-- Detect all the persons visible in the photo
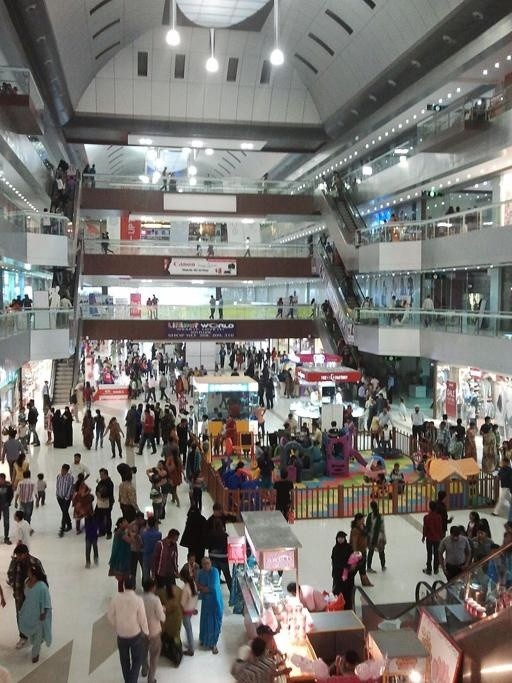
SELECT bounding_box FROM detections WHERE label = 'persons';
[244,236,252,256]
[207,236,215,257]
[309,298,350,362]
[7,294,33,326]
[361,297,407,325]
[160,166,170,192]
[195,233,203,255]
[307,158,486,297]
[147,294,159,320]
[209,295,223,320]
[1,340,511,682]
[260,171,269,194]
[60,291,73,324]
[421,294,434,327]
[2,77,114,287]
[275,295,294,318]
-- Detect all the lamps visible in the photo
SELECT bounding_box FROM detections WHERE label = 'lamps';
[203,26,222,74]
[268,0,286,67]
[162,0,184,48]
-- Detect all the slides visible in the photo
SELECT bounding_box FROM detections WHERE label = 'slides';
[351,449,367,468]
